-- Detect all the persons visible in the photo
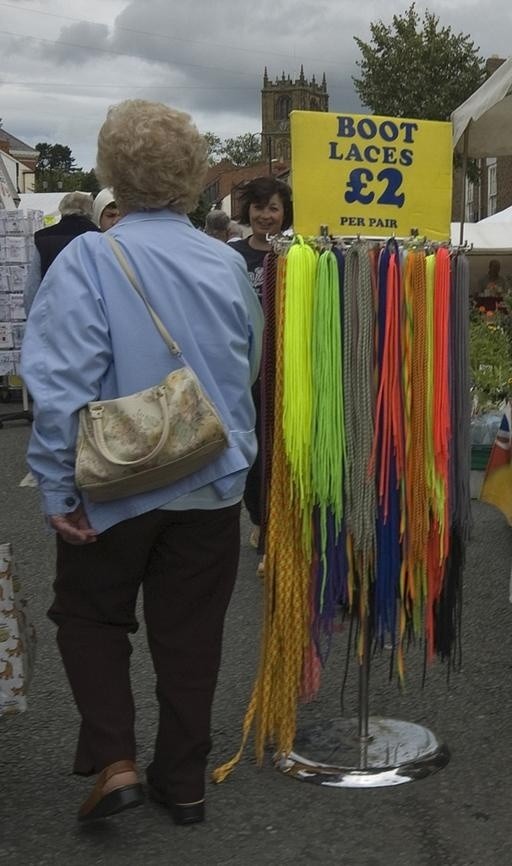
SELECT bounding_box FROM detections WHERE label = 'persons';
[187,195,243,246]
[223,174,297,581]
[473,258,505,305]
[89,186,120,232]
[16,96,260,833]
[22,192,100,320]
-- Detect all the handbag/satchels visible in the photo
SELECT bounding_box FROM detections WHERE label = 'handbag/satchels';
[75,368,229,506]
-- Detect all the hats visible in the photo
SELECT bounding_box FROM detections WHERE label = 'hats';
[92,187,115,229]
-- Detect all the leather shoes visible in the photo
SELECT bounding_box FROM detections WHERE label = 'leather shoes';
[137,770,207,826]
[78,760,144,823]
